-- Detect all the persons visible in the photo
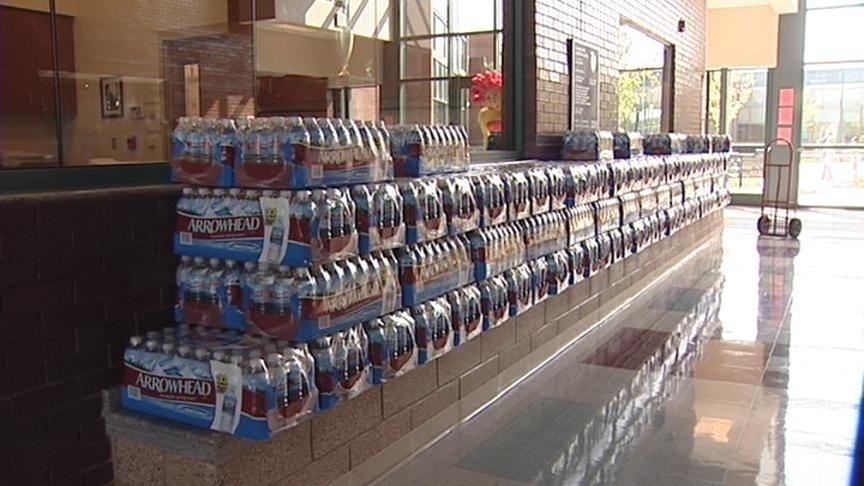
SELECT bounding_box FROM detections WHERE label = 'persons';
[470,67,502,150]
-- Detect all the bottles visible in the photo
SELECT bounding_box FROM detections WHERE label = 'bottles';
[122,116,731,440]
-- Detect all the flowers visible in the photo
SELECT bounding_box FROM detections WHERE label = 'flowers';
[470,70,502,105]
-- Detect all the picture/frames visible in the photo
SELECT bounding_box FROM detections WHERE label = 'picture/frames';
[181,60,202,117]
[570,38,601,133]
[101,76,124,118]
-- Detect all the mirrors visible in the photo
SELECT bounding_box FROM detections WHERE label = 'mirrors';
[0,0,256,170]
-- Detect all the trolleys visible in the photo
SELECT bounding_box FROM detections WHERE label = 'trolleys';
[756,136,802,242]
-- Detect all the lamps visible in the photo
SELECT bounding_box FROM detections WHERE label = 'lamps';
[334,0,355,77]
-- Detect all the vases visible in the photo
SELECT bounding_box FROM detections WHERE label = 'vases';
[478,105,501,149]
